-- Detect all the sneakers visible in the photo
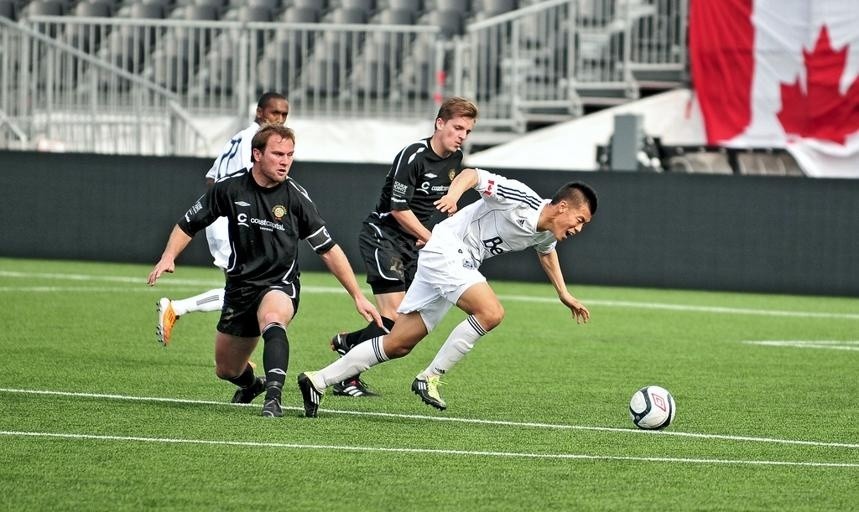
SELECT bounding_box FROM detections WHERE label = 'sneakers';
[261,397,284,418]
[229,376,267,404]
[156,297,180,348]
[329,331,351,359]
[297,371,324,419]
[332,378,381,397]
[411,369,447,413]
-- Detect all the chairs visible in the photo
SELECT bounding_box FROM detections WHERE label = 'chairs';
[0,1,693,152]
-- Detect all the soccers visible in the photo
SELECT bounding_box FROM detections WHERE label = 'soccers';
[628,386,675,430]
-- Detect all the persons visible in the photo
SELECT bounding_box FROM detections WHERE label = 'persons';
[298,167,597,416]
[148,124,383,418]
[330,97,477,397]
[157,92,288,347]
